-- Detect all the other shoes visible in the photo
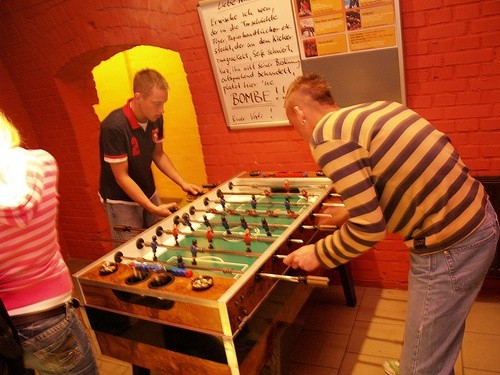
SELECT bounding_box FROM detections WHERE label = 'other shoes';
[383,360,400,375]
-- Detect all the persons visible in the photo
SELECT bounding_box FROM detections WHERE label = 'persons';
[0,110,102,375]
[282,72,500,375]
[98,68,203,249]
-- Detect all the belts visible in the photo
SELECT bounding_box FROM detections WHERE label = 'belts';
[10,297,81,327]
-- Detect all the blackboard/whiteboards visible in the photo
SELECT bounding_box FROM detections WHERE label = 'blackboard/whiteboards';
[199,1,308,126]
